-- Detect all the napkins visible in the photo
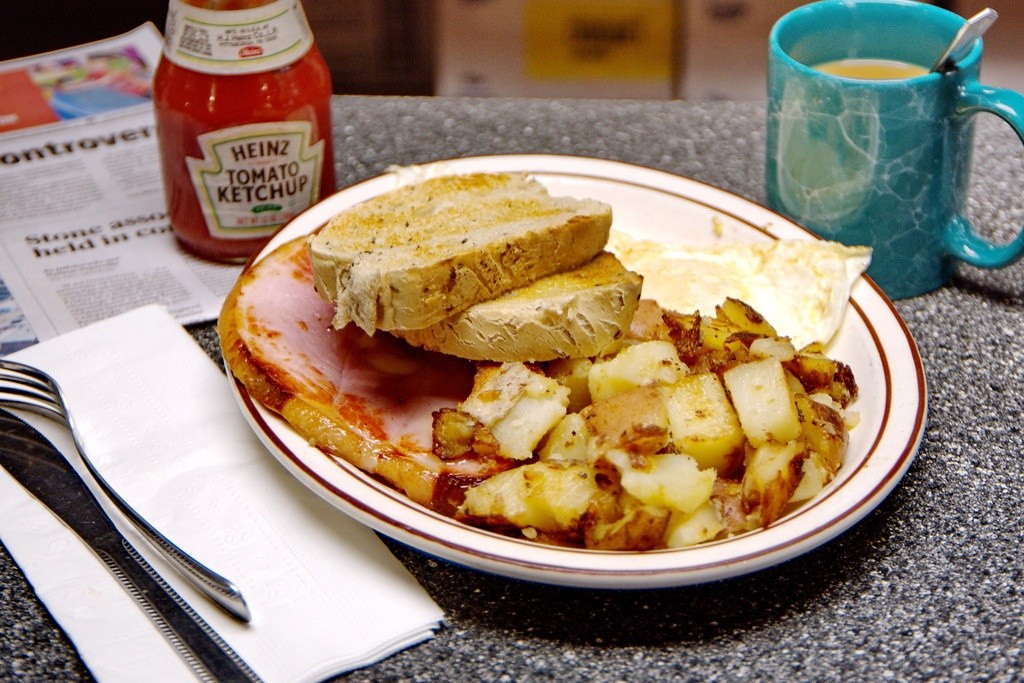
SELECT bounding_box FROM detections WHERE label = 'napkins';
[0,303,448,683]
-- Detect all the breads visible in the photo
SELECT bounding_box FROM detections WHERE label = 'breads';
[307,169,644,363]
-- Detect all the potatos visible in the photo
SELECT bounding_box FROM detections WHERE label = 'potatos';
[434,294,855,554]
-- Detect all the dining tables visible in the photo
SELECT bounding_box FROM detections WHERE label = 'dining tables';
[0,94,1024,683]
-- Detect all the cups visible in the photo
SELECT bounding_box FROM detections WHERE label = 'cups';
[761,1,1024,301]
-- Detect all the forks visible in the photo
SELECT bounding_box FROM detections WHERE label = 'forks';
[0,360,252,625]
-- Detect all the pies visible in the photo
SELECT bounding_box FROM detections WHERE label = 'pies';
[595,230,873,353]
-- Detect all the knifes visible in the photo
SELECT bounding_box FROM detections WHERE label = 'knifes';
[0,407,264,683]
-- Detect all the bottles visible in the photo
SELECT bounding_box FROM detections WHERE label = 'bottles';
[150,0,336,268]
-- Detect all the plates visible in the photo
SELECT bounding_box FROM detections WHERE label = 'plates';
[214,151,934,586]
